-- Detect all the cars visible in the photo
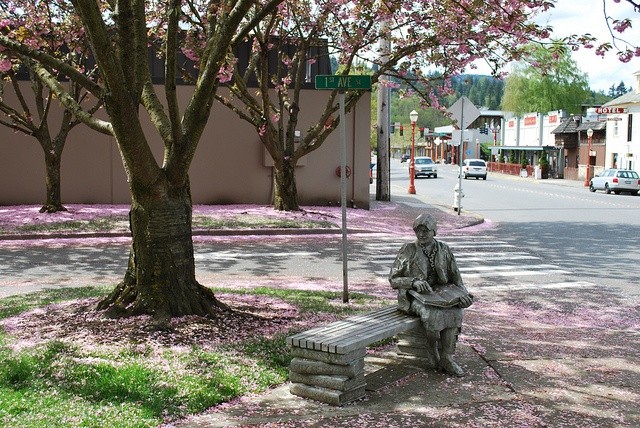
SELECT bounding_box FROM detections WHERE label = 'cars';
[590,168,640,195]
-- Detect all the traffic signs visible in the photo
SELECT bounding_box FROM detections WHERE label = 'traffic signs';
[315,74,373,90]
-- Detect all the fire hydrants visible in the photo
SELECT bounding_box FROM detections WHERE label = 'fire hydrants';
[452,183,465,212]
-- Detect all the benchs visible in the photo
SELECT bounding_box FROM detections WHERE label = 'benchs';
[287,304,443,407]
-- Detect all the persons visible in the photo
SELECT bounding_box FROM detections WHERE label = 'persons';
[389,212,474,378]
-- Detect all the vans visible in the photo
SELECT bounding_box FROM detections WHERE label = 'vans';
[457,157,488,179]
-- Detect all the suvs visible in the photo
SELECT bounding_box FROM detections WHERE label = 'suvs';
[408,156,438,179]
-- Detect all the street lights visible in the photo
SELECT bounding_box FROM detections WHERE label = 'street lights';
[408,109,419,195]
[584,127,594,187]
[490,124,501,162]
[476,138,480,159]
